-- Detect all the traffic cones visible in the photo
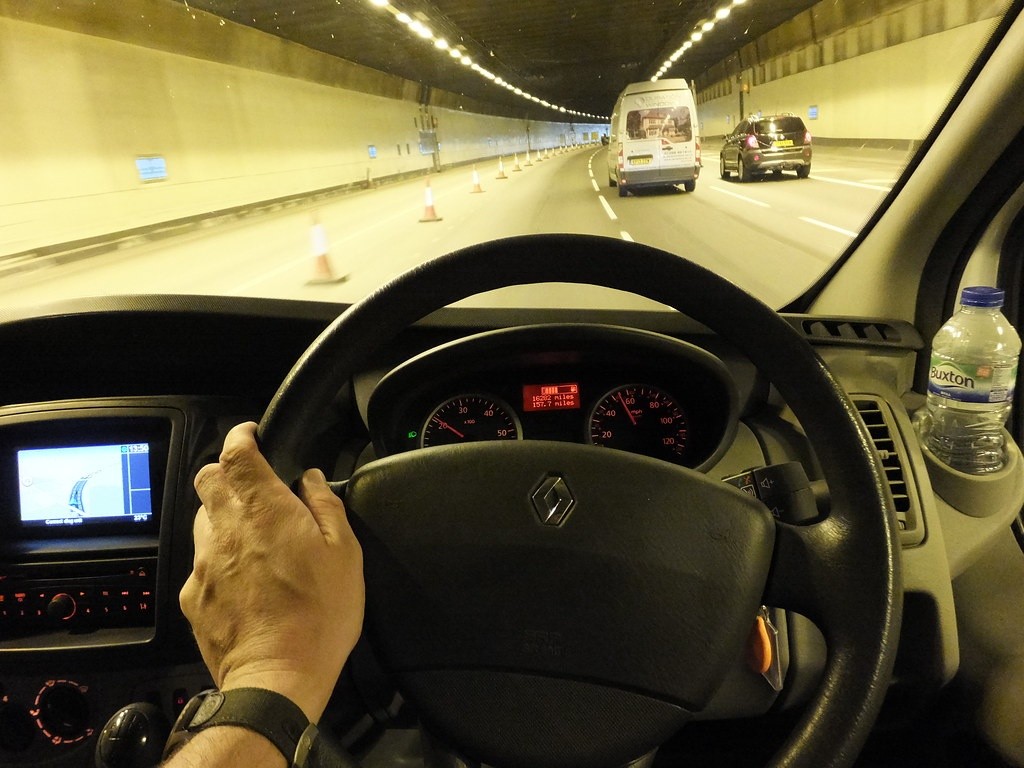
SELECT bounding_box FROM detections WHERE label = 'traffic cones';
[525,149,533,167]
[552,148,556,157]
[558,145,563,153]
[536,149,543,162]
[418,175,444,223]
[564,144,568,153]
[570,143,574,151]
[496,157,507,179]
[546,149,550,159]
[575,139,601,150]
[469,164,485,193]
[513,153,522,171]
[305,215,349,285]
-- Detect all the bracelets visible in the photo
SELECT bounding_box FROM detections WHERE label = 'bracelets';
[161,688,323,768]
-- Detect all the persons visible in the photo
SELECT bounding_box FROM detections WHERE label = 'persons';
[160,423,366,768]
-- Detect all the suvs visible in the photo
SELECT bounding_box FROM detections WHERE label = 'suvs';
[719,113,812,180]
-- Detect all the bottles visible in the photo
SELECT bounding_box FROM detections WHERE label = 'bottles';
[922,286,1022,475]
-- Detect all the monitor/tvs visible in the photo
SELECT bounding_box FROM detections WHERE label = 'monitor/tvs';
[16,443,153,529]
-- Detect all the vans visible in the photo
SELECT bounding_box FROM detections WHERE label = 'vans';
[600,78,700,197]
